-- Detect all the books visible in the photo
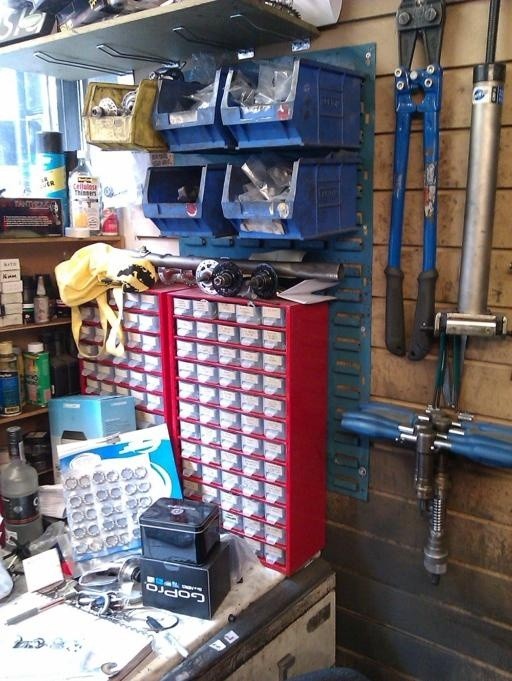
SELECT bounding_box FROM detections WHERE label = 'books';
[0,591,153,681]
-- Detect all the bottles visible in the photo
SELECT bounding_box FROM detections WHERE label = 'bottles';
[0,341,23,416]
[23,342,51,407]
[35,132,68,234]
[101,209,119,236]
[69,150,100,236]
[0,425,44,550]
[33,276,50,324]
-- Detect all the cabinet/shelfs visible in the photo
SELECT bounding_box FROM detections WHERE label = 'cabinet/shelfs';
[166,287,329,579]
[74,285,186,432]
[0,233,126,446]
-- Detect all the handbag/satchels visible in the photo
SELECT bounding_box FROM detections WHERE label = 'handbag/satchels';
[54,243,157,307]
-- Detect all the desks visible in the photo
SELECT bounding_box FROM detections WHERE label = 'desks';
[0,506,339,681]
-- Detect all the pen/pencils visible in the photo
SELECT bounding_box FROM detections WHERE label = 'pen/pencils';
[146,616,189,659]
[7,592,75,624]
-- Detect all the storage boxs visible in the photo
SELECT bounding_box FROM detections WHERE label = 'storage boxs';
[220,156,360,240]
[47,394,137,484]
[220,57,366,152]
[149,66,233,154]
[140,163,232,237]
[82,79,169,154]
